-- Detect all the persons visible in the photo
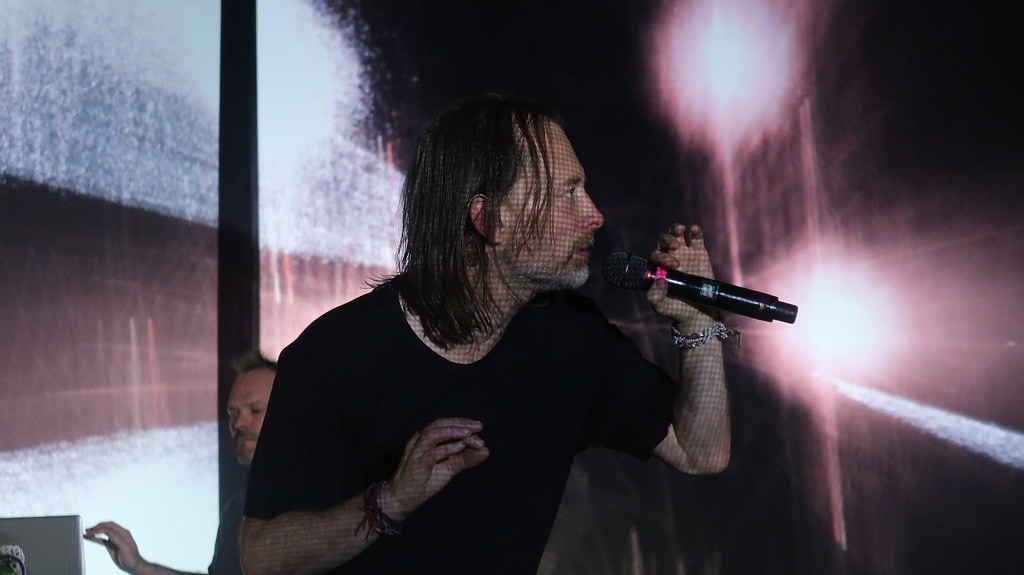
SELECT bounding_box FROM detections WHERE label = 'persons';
[84,351,277,575]
[236,92,733,575]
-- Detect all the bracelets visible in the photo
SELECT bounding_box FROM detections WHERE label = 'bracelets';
[353,477,404,540]
[669,320,741,351]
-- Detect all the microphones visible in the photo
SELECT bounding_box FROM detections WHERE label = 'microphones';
[601,253,799,324]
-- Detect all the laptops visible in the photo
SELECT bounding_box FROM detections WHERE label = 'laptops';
[0,515,87,575]
